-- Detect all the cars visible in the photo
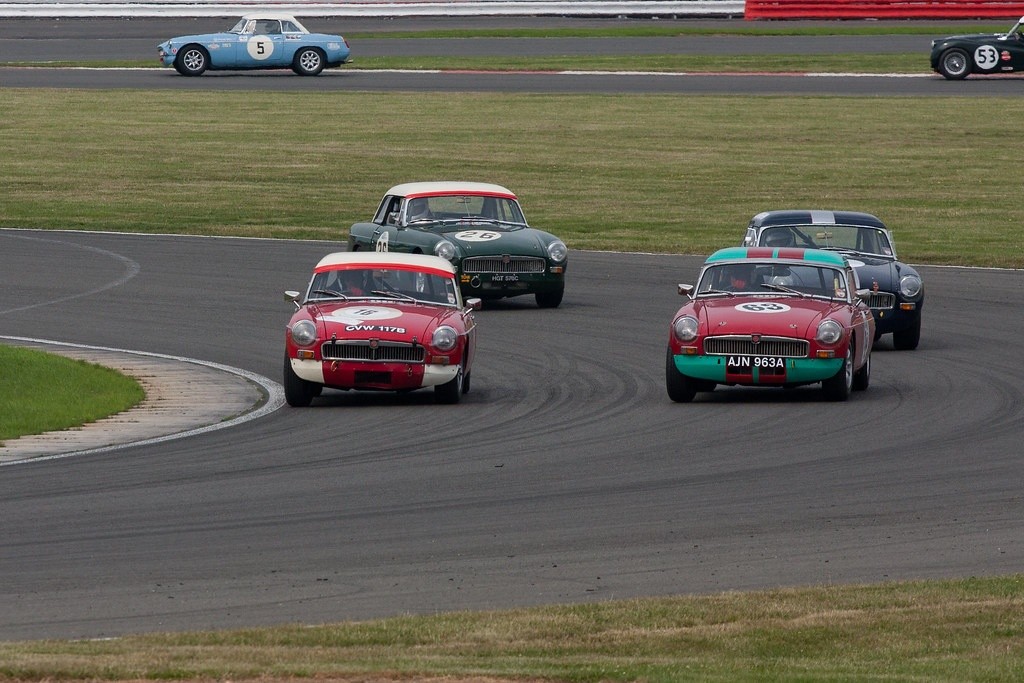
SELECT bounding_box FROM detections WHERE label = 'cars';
[930,15,1024,81]
[665,246,876,402]
[740,210,924,352]
[282,251,482,408]
[347,180,569,307]
[158,11,351,77]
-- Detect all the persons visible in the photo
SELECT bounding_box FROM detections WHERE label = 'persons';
[398,197,437,225]
[718,263,758,295]
[263,21,279,33]
[333,269,375,298]
[764,227,798,249]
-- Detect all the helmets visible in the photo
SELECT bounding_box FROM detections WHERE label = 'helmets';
[265,23,278,32]
[337,270,368,290]
[408,197,429,220]
[724,265,757,289]
[765,227,795,247]
